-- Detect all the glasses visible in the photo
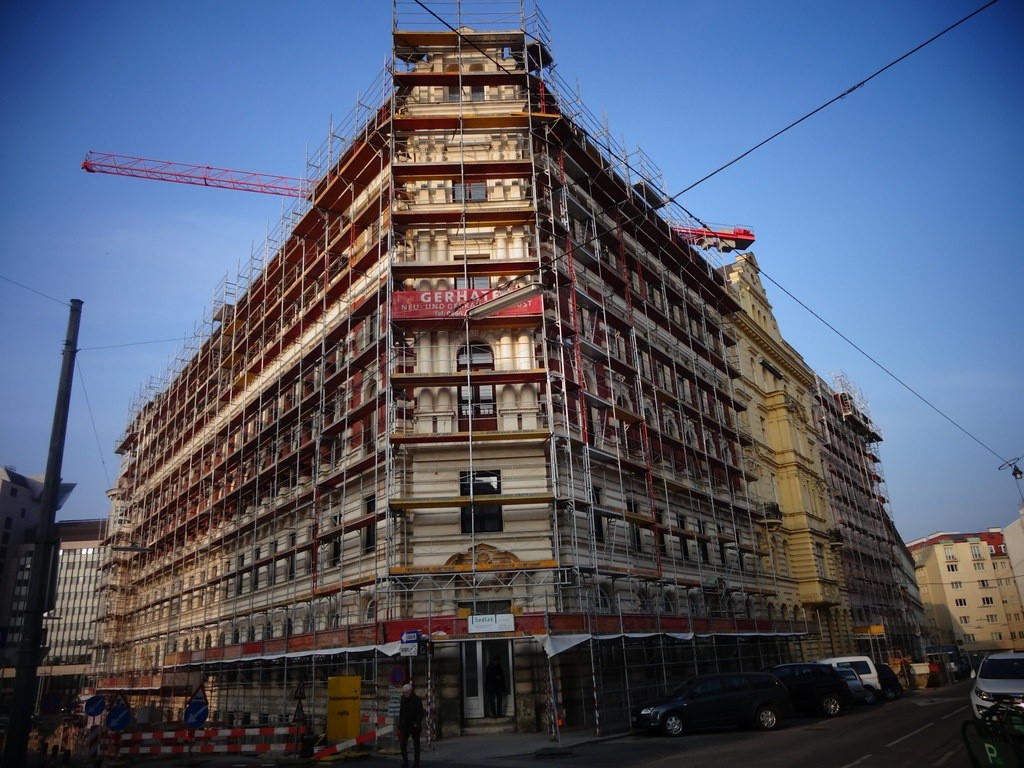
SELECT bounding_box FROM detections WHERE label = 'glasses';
[403,692,409,694]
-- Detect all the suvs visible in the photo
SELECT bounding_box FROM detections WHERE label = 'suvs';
[969,652,1024,721]
[775,664,854,718]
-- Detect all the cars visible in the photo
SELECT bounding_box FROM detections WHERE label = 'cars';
[874,663,903,702]
[836,668,864,690]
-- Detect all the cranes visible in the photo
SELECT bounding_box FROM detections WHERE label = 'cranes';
[81,150,756,253]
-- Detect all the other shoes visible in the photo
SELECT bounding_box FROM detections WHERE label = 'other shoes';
[399,763,409,768]
[413,763,419,768]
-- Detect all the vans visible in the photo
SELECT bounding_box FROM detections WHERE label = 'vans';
[820,656,881,705]
[631,673,795,737]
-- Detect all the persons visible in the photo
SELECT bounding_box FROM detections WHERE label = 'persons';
[396,684,426,768]
[486,654,506,719]
